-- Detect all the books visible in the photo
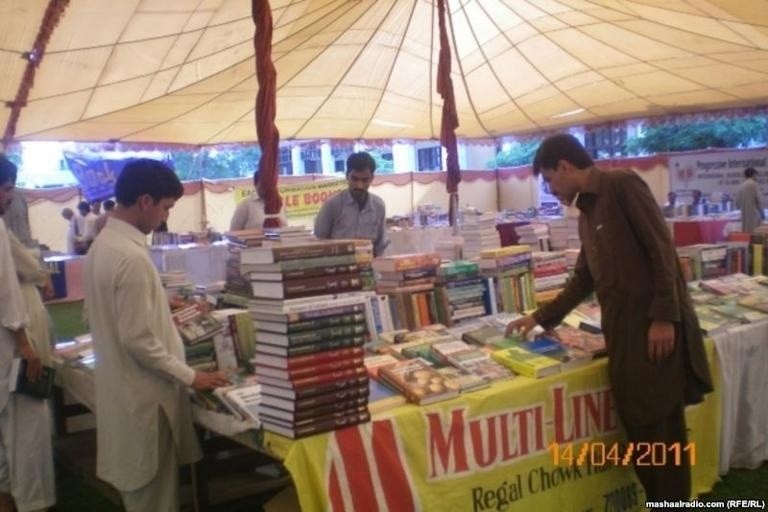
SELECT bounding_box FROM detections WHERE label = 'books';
[7,357,56,400]
[375,215,606,410]
[169,222,314,427]
[675,241,768,338]
[150,226,224,245]
[239,237,374,440]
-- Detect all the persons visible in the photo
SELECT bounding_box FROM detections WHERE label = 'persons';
[0,152,58,512]
[664,191,679,207]
[60,206,83,253]
[76,200,98,248]
[94,199,116,237]
[229,170,287,232]
[312,151,390,257]
[91,198,100,214]
[505,133,717,512]
[690,189,703,206]
[735,166,766,232]
[80,158,235,511]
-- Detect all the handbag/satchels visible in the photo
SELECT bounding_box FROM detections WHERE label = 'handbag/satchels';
[74,218,87,253]
